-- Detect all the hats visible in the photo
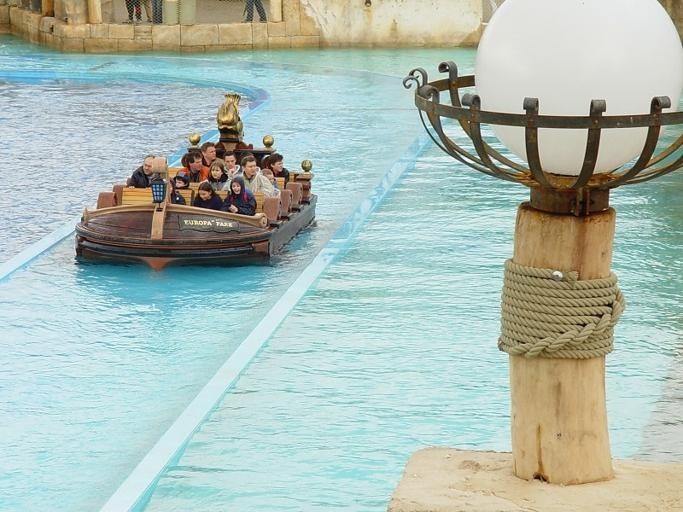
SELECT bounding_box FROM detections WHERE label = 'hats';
[174,171,189,187]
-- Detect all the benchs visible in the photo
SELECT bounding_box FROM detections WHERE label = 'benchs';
[122,168,294,213]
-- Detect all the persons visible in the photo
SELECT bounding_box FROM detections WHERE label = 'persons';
[170,142,290,217]
[124,0,154,25]
[125,154,156,189]
[240,0,267,24]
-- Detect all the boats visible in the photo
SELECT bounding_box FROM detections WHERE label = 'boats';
[70,145,322,269]
